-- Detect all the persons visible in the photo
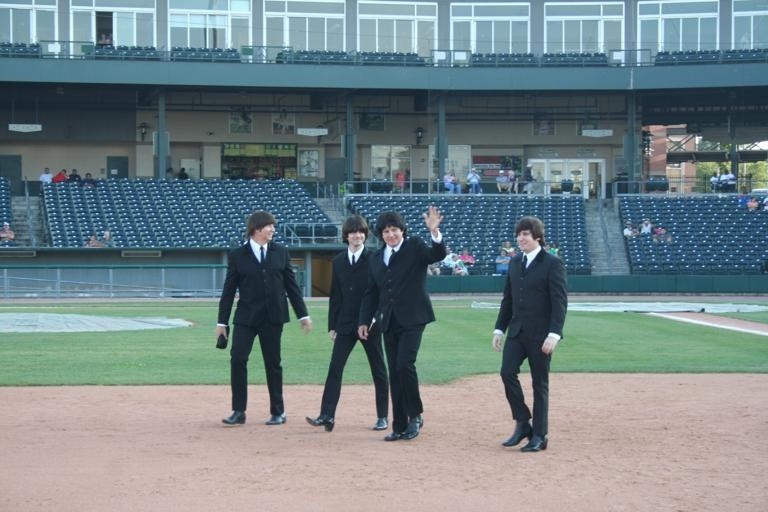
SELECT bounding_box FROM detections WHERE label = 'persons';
[623,218,672,242]
[542,237,560,256]
[492,217,567,452]
[84,236,100,247]
[739,191,768,212]
[179,167,188,179]
[496,170,511,193]
[710,169,736,193]
[466,167,482,194]
[102,232,114,247]
[495,250,511,275]
[426,244,475,276]
[358,205,447,440]
[503,240,515,256]
[305,215,389,431]
[0,222,15,247]
[166,168,175,177]
[444,169,462,194]
[39,168,96,196]
[508,171,519,194]
[215,210,312,423]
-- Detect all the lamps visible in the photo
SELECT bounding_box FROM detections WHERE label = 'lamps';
[412,125,428,146]
[136,121,152,142]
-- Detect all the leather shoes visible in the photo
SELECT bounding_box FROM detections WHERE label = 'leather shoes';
[306,417,333,431]
[374,415,423,441]
[223,412,245,424]
[503,424,546,451]
[266,415,286,425]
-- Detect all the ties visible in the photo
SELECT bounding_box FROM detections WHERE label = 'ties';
[352,255,354,264]
[260,247,264,262]
[521,255,527,272]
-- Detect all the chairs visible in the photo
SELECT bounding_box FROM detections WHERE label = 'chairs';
[473,51,611,68]
[343,194,593,277]
[39,175,342,247]
[81,43,163,61]
[655,47,766,65]
[1,176,18,247]
[1,40,43,59]
[277,51,428,67]
[170,46,242,63]
[618,191,767,278]
